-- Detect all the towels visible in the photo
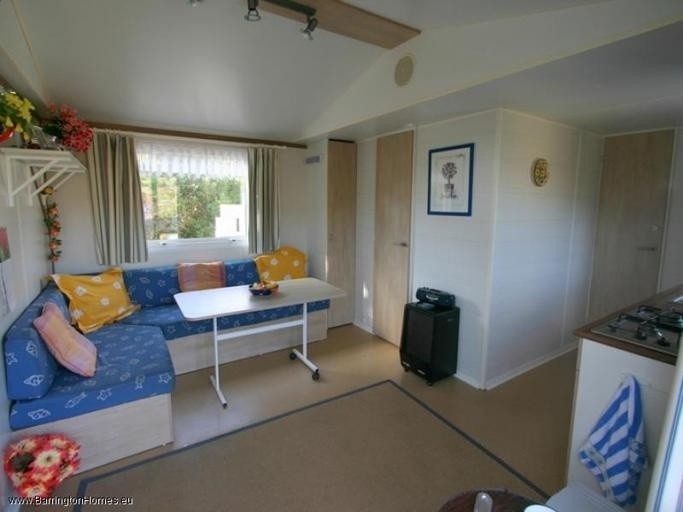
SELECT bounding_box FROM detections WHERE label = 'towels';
[576,375,650,508]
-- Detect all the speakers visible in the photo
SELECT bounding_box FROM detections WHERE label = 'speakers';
[399,302,460,386]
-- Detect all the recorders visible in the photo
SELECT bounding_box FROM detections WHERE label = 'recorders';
[415,287,455,305]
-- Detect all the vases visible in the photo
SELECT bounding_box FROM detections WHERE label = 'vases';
[47,133,63,151]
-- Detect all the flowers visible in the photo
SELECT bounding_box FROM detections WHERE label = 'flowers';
[42,103,93,151]
[3,433,81,500]
[0,86,35,153]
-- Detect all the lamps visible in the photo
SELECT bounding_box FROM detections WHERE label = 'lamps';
[243,0,318,41]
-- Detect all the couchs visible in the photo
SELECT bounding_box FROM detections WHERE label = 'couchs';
[2,247,330,480]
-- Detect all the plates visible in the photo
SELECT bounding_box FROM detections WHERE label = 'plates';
[249,284,279,296]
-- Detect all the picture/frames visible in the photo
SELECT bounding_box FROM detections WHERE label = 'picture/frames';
[427,142,474,216]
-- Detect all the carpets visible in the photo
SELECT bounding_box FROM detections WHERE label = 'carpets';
[72,379,550,512]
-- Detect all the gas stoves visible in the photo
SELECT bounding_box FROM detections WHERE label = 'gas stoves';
[590,303,683,357]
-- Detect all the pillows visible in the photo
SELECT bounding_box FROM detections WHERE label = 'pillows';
[40,264,142,334]
[255,246,306,283]
[177,261,226,291]
[32,302,97,377]
